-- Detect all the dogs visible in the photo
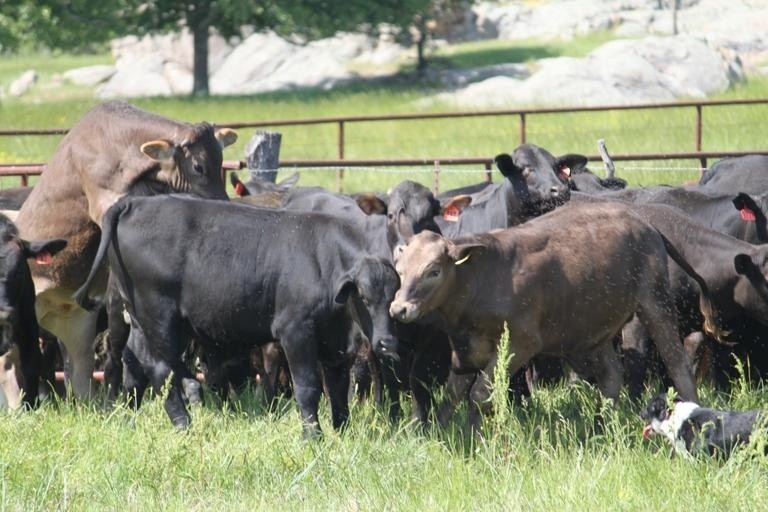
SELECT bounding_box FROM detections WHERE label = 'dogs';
[641,395,768,467]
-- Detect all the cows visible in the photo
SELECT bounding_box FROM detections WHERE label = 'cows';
[0,100,766,453]
